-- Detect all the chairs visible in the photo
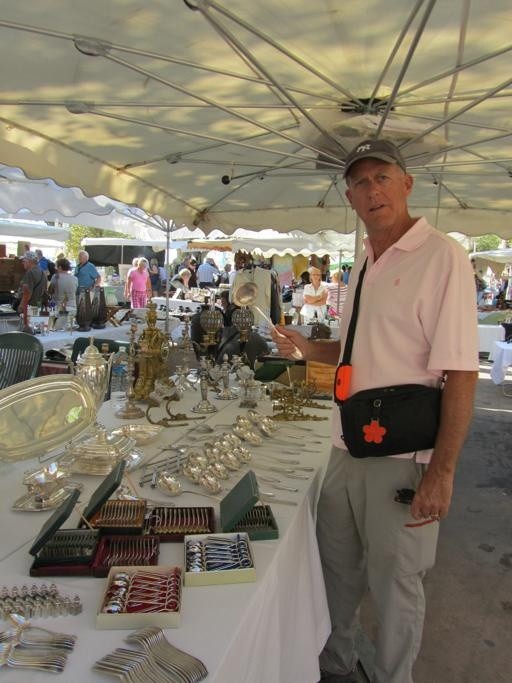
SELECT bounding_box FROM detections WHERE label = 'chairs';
[0,328,45,392]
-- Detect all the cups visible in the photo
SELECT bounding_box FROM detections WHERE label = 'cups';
[192,287,200,294]
[40,324,48,336]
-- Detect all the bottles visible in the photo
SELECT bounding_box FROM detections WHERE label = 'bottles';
[17,314,24,330]
[110,345,130,407]
[113,269,119,280]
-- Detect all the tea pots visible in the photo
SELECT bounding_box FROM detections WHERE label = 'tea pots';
[72,335,113,432]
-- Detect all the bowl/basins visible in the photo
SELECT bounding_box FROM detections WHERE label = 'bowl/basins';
[111,422,166,446]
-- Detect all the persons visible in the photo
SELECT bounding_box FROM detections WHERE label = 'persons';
[290,255,352,327]
[470,258,484,307]
[269,140,480,682]
[12,248,243,314]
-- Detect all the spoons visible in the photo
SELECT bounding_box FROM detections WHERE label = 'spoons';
[0,612,76,675]
[153,410,329,501]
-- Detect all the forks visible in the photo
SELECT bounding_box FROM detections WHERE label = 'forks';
[126,627,211,681]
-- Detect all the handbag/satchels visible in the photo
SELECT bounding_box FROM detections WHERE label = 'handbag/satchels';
[327,380,471,458]
[15,289,30,311]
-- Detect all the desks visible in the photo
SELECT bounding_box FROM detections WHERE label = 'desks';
[149,295,205,313]
[281,322,341,338]
[0,364,335,682]
[98,283,129,305]
[0,312,183,355]
[489,340,512,398]
[477,324,507,356]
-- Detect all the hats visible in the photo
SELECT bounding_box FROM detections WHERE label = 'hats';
[333,130,413,173]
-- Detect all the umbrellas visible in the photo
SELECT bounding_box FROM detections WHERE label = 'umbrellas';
[0,0,512,263]
[231,229,372,318]
[1,163,253,335]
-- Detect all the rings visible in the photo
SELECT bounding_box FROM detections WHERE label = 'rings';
[430,514,439,518]
